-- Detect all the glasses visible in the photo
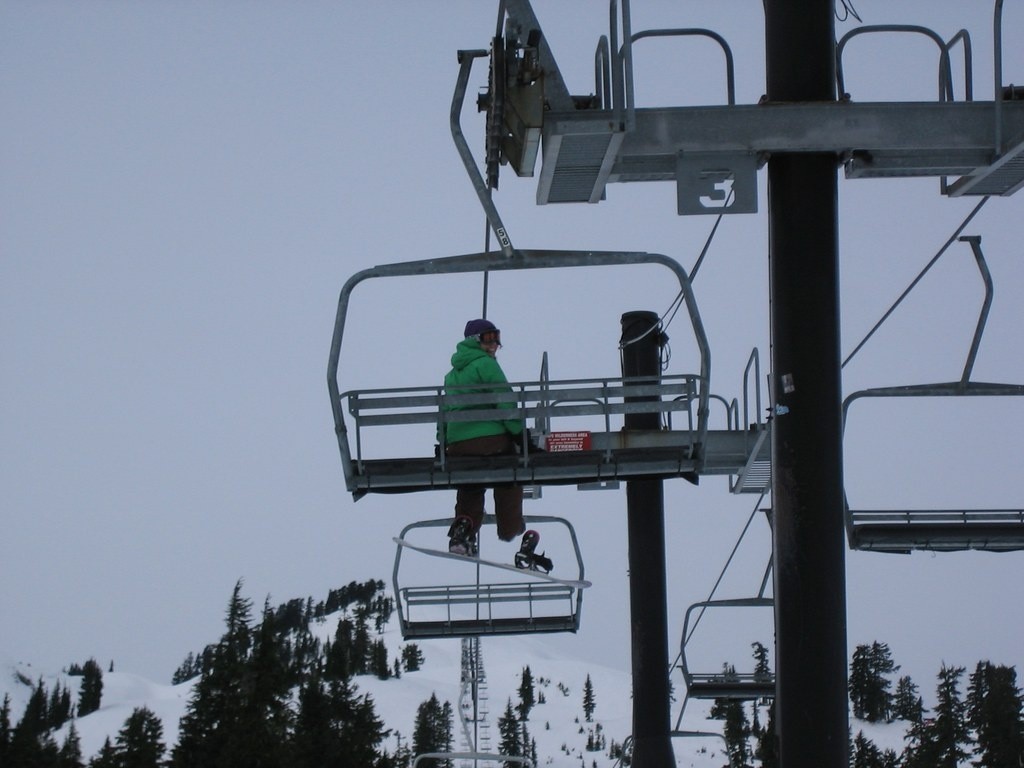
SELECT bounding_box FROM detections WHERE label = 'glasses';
[480,340,501,345]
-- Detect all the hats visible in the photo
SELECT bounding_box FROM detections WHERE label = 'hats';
[464,319,500,337]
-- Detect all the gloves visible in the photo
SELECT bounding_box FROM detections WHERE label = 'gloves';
[434,443,447,455]
[513,430,537,454]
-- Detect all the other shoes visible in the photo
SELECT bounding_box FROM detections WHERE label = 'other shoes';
[499,519,526,542]
[447,522,477,557]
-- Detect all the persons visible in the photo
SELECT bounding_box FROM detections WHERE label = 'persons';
[436,319,526,555]
